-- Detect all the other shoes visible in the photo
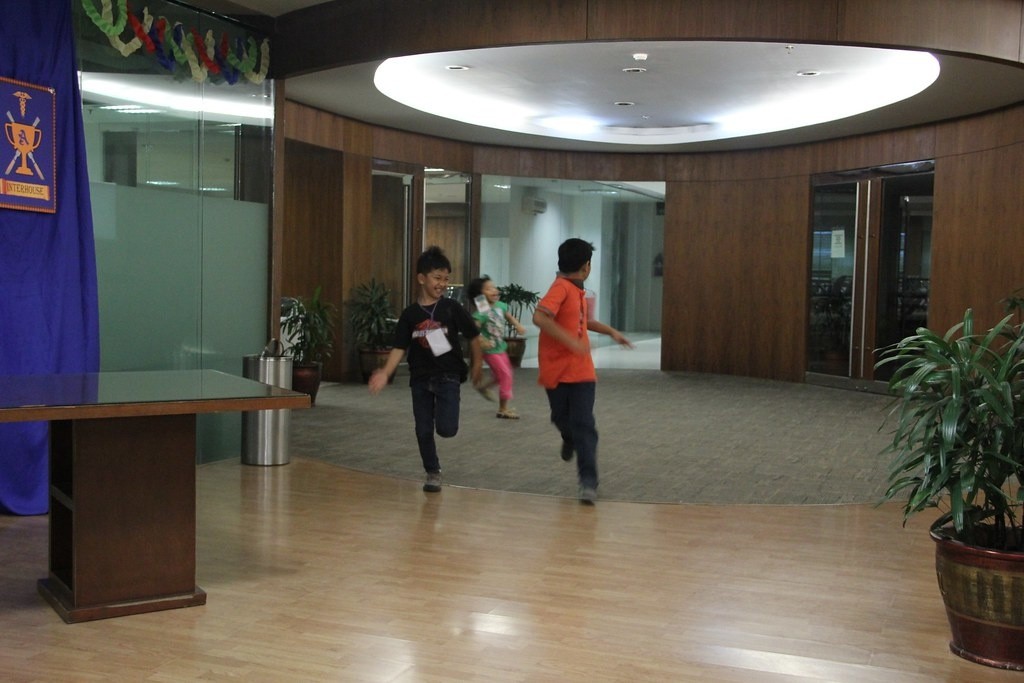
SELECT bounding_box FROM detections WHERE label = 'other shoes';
[480,388,496,400]
[561,441,573,460]
[578,482,596,500]
[423,470,442,491]
[497,410,520,419]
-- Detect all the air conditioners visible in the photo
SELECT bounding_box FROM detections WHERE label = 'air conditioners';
[521,196,547,216]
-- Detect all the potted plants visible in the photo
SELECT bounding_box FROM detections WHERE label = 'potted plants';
[281,272,345,408]
[345,278,397,384]
[865,307,1024,672]
[497,283,542,368]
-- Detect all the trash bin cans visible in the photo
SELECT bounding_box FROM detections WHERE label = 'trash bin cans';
[241,338,293,467]
[281,298,305,359]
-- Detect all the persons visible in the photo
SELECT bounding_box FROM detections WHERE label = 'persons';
[467,274,528,419]
[367,246,483,492]
[532,238,636,506]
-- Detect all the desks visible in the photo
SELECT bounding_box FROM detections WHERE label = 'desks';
[0,367,313,625]
